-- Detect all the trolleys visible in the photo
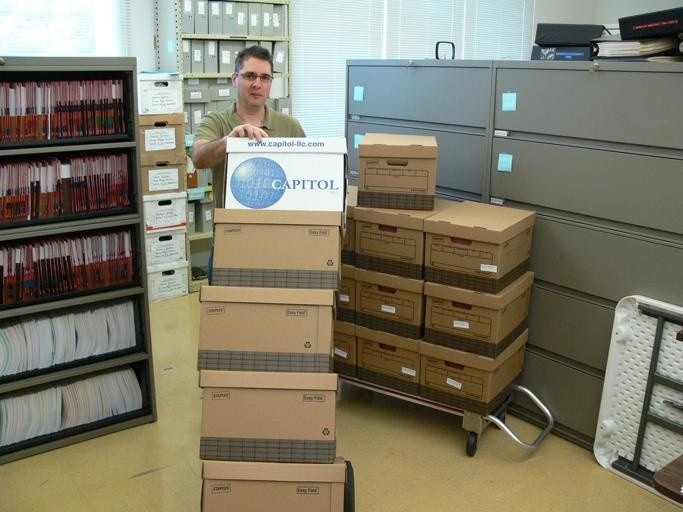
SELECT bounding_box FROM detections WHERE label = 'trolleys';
[335,373,555,456]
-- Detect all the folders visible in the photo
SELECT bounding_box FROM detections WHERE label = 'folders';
[531,23,612,62]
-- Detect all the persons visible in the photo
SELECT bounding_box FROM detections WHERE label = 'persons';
[190,46,306,285]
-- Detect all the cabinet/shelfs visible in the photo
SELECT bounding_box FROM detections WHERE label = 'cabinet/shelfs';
[154,0,287,301]
[0,59,155,461]
[493,61,683,453]
[343,55,495,203]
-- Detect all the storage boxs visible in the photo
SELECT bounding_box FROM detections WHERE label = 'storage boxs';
[422,271,536,358]
[134,71,193,298]
[357,325,422,394]
[356,133,438,212]
[212,207,345,294]
[418,327,530,417]
[335,321,357,378]
[338,186,358,265]
[198,370,338,462]
[355,199,458,280]
[224,136,348,213]
[199,464,346,512]
[196,286,338,373]
[338,263,425,340]
[423,200,535,295]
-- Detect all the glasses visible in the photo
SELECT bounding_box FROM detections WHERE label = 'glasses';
[236,72,272,81]
